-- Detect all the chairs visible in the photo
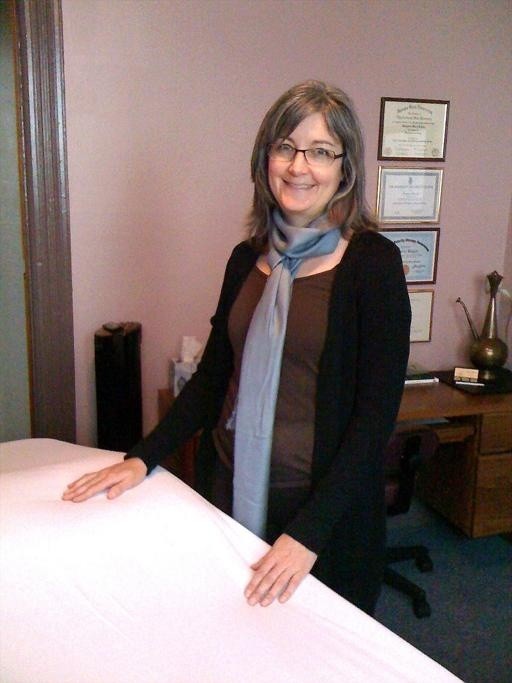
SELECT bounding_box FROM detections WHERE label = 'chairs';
[379,424,439,618]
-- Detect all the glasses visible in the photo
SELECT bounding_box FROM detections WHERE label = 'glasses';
[265,141,347,167]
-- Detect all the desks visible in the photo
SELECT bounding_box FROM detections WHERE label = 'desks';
[157,364,512,539]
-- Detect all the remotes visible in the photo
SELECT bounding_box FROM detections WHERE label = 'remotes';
[103,322,123,333]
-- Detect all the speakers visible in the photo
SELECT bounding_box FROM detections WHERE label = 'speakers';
[94,321,143,453]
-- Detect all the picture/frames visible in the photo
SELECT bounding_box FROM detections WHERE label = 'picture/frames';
[374,94,450,344]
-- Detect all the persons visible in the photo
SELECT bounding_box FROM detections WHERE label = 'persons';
[62,79,412,618]
